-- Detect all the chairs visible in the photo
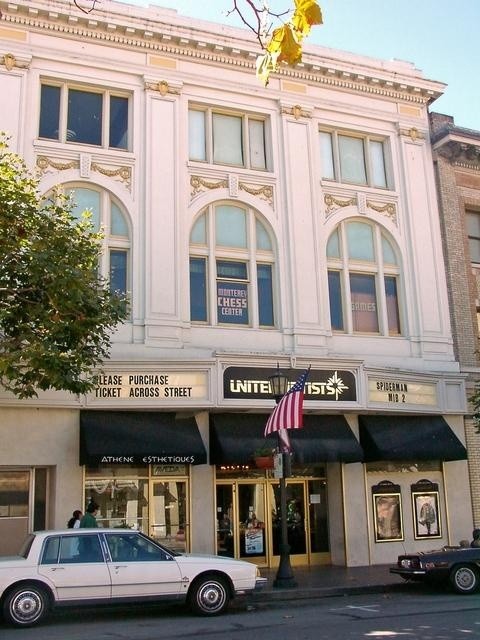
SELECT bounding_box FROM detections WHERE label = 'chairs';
[460,540,469,548]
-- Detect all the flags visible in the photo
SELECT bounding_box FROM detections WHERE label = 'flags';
[264,367,314,439]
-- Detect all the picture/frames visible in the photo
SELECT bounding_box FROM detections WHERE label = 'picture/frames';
[410,479,443,540]
[372,480,404,542]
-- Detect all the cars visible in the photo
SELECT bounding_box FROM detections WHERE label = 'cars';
[0,529,268,628]
[389,538,480,595]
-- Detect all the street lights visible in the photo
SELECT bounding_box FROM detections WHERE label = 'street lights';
[268,361,300,589]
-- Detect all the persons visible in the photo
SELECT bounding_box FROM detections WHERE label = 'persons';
[81,502,102,529]
[68,511,83,558]
